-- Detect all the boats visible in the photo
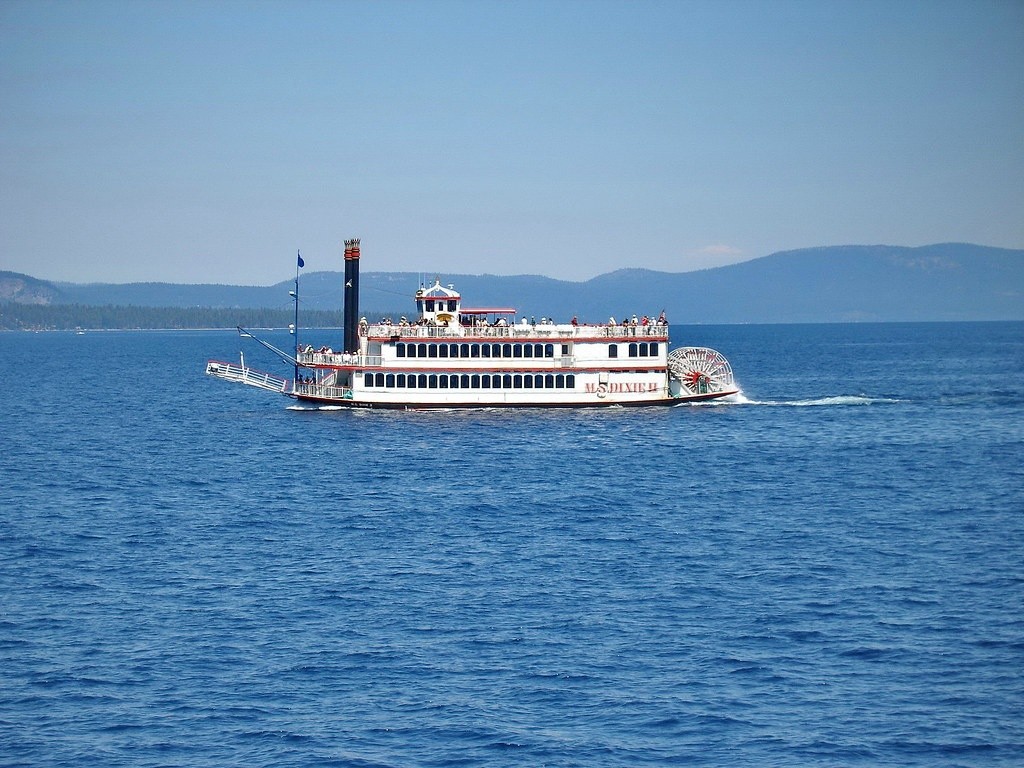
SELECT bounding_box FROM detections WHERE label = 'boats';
[205,237,739,409]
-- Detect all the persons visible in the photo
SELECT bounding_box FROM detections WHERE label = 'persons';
[359,314,554,337]
[572,316,578,325]
[609,314,669,326]
[297,344,358,365]
[298,374,316,394]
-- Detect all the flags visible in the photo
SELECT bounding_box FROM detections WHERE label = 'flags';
[659,309,666,320]
[298,253,304,267]
